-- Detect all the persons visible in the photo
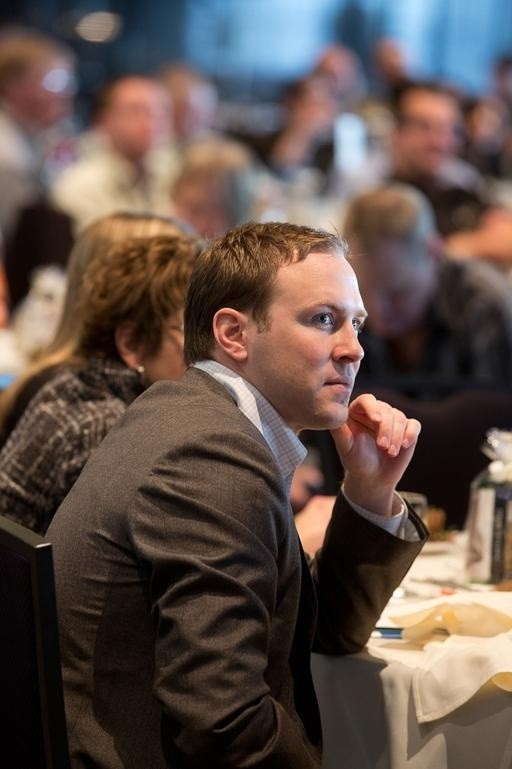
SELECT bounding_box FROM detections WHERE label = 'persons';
[1,26,510,543]
[40,220,433,769]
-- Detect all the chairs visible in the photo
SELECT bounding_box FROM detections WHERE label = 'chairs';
[0,515,71,769]
